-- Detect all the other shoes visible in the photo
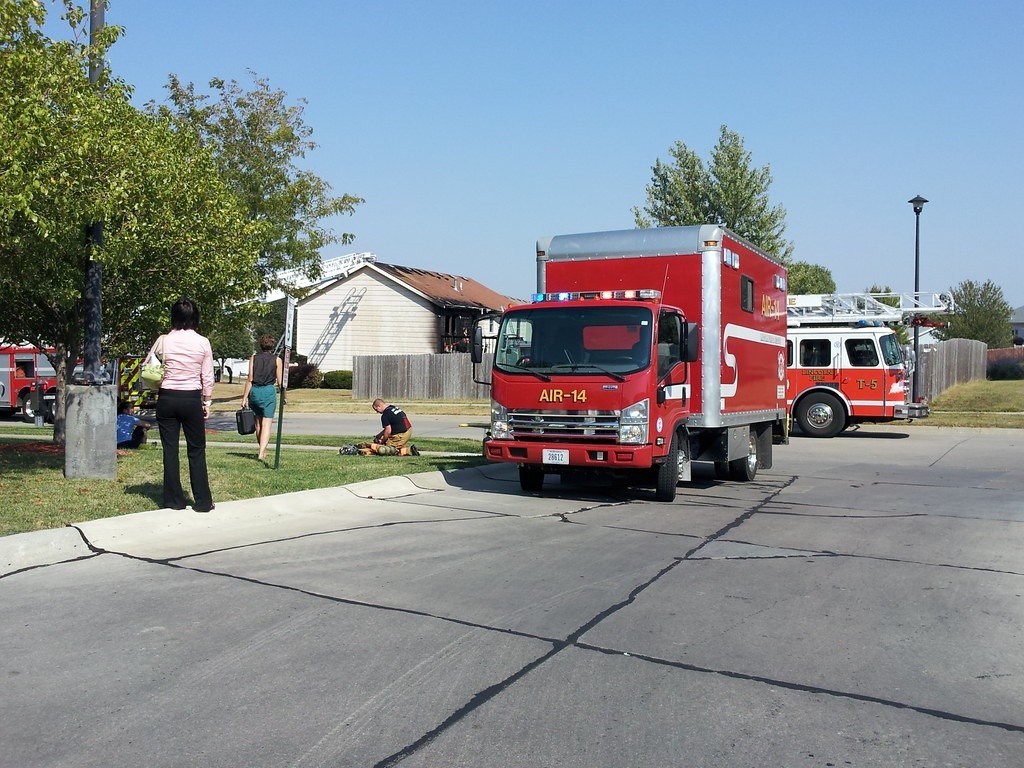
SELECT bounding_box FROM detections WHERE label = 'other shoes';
[410,444,420,456]
[258,456,268,467]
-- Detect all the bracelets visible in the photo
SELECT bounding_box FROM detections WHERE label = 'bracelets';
[202,399,213,405]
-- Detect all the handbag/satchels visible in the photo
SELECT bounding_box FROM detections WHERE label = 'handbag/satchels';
[236,405,256,435]
[140,335,166,391]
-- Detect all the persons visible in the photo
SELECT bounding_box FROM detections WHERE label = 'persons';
[241,335,287,461]
[142,298,215,512]
[215,366,232,383]
[372,398,420,456]
[117,404,150,449]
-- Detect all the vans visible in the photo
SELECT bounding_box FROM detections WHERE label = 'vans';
[101,352,159,408]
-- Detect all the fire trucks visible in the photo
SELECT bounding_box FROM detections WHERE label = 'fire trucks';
[468,222,789,502]
[0,344,103,425]
[786,288,956,438]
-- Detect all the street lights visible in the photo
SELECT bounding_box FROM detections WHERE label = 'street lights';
[908,195,930,404]
